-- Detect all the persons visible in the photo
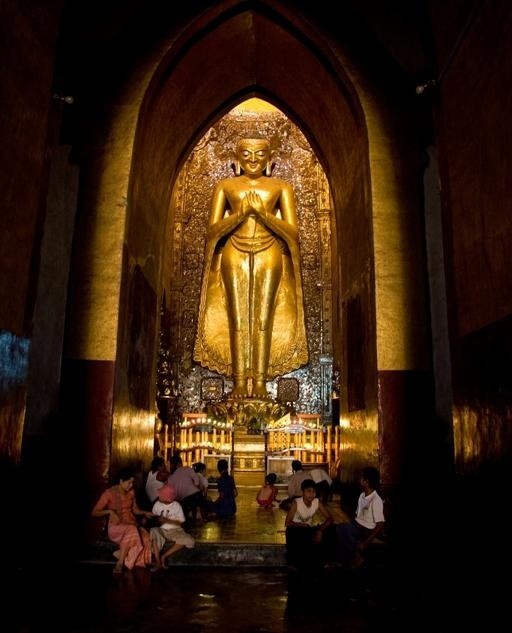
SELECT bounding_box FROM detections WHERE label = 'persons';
[192,132,309,400]
[257,460,386,572]
[91,454,238,580]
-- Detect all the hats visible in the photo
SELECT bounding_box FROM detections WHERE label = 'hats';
[157,484,178,502]
